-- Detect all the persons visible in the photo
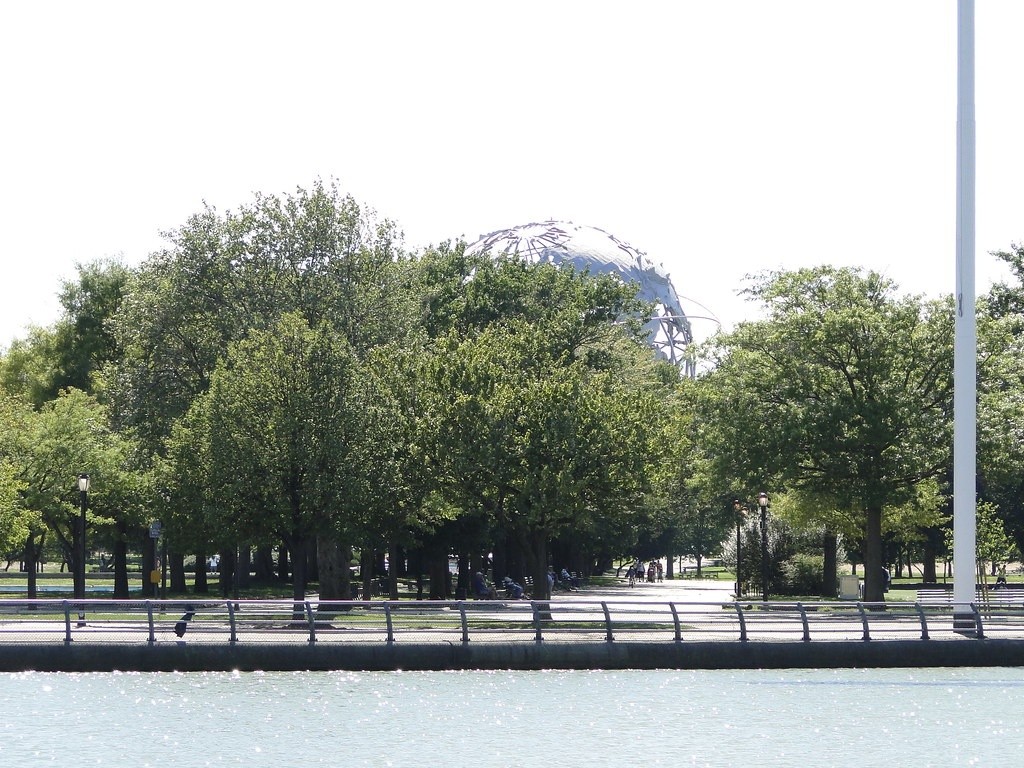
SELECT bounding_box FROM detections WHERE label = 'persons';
[476,572,524,601]
[992,564,1008,591]
[625,558,663,586]
[157,557,160,571]
[547,566,579,596]
[101,554,107,572]
[208,555,219,574]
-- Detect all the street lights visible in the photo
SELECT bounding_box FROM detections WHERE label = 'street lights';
[75,473,91,627]
[733,500,748,598]
[759,492,770,602]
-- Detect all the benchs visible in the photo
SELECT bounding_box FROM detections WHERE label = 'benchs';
[917,588,1024,618]
[490,571,588,598]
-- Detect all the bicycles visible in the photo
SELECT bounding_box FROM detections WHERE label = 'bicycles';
[630,577,636,588]
